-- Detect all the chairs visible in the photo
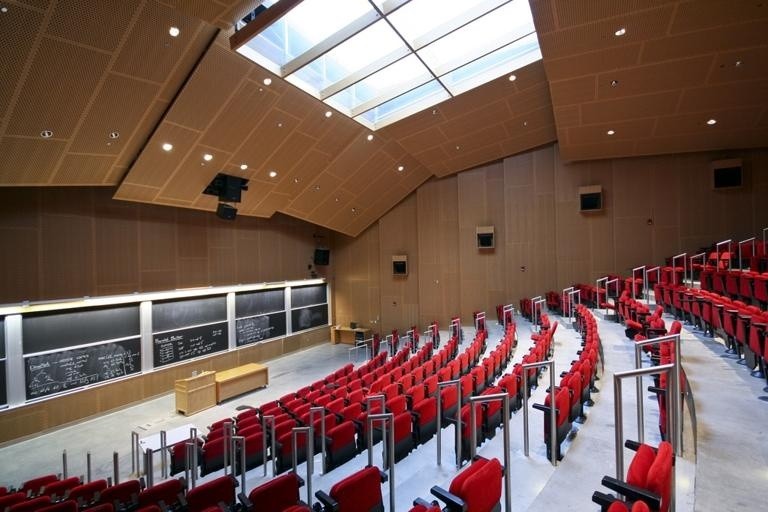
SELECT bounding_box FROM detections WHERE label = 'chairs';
[471,366,486,395]
[561,359,591,418]
[475,336,482,354]
[431,320,439,342]
[496,374,519,419]
[591,491,649,512]
[531,386,570,463]
[11,496,51,512]
[753,273,768,305]
[443,344,451,362]
[540,320,551,330]
[236,424,262,437]
[669,286,685,309]
[321,383,335,394]
[358,365,368,378]
[437,366,452,383]
[661,283,672,306]
[413,396,441,441]
[233,416,260,430]
[69,477,112,502]
[447,359,461,381]
[439,384,462,422]
[426,342,433,357]
[725,270,742,297]
[325,374,336,383]
[361,372,374,387]
[544,290,570,312]
[538,317,548,324]
[599,290,650,333]
[371,333,380,354]
[572,349,595,389]
[260,406,284,422]
[302,389,321,403]
[167,441,201,477]
[490,350,501,377]
[390,328,399,350]
[295,386,311,397]
[377,373,391,387]
[276,391,297,406]
[496,343,507,368]
[383,362,393,374]
[348,378,362,391]
[600,274,644,298]
[35,500,78,512]
[259,400,278,414]
[458,352,469,376]
[375,366,384,380]
[578,340,598,376]
[202,427,228,443]
[335,368,345,379]
[717,239,766,258]
[382,383,398,400]
[397,351,405,365]
[483,356,494,385]
[479,385,501,431]
[506,325,515,330]
[347,371,359,380]
[521,354,537,386]
[313,413,339,433]
[390,367,402,381]
[582,327,597,336]
[134,504,162,512]
[311,393,331,408]
[549,371,584,423]
[533,340,546,362]
[601,439,673,512]
[380,351,387,365]
[296,407,326,425]
[0,493,26,507]
[394,373,413,393]
[452,336,459,355]
[283,398,306,412]
[736,305,760,346]
[445,401,483,451]
[323,420,355,465]
[361,393,382,410]
[696,293,719,326]
[206,417,235,431]
[344,388,363,404]
[667,251,733,269]
[451,315,461,336]
[582,332,598,341]
[479,332,484,348]
[331,385,348,398]
[711,270,725,293]
[504,333,514,349]
[573,304,597,327]
[366,378,383,395]
[749,311,767,357]
[738,271,759,300]
[267,413,289,427]
[137,476,188,512]
[378,374,391,385]
[417,350,424,366]
[0,486,7,497]
[270,419,300,439]
[653,282,664,302]
[45,474,84,496]
[537,334,551,362]
[335,376,348,388]
[186,473,239,512]
[686,290,709,317]
[347,379,362,390]
[471,341,480,361]
[411,355,419,370]
[402,361,412,374]
[633,264,683,285]
[505,328,514,337]
[540,314,547,320]
[101,477,145,505]
[24,473,62,495]
[323,397,344,416]
[573,283,606,302]
[700,268,712,291]
[423,373,439,398]
[621,306,685,440]
[289,402,314,417]
[505,365,529,402]
[422,346,429,361]
[334,402,363,424]
[199,435,234,478]
[460,374,473,405]
[530,321,558,344]
[405,324,419,347]
[237,432,270,470]
[368,359,375,372]
[391,355,400,367]
[402,383,426,407]
[500,338,510,360]
[678,288,697,314]
[374,355,381,367]
[237,471,305,512]
[519,298,542,317]
[722,300,743,338]
[710,296,731,329]
[432,354,441,373]
[403,348,409,362]
[81,503,114,512]
[507,322,515,326]
[352,405,382,450]
[527,345,543,374]
[448,340,456,359]
[424,359,433,378]
[312,380,324,390]
[278,430,307,467]
[233,407,258,424]
[373,410,412,469]
[430,454,504,512]
[465,345,475,368]
[344,363,353,375]
[315,464,388,512]
[473,311,486,330]
[439,349,447,367]
[411,366,424,386]
[496,304,512,324]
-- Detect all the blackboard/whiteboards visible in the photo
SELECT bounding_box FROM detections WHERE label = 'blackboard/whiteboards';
[226,312,287,347]
[152,292,228,335]
[0,316,7,360]
[0,359,8,406]
[234,286,286,323]
[22,302,141,357]
[290,282,327,311]
[14,336,141,401]
[291,304,329,333]
[138,322,230,368]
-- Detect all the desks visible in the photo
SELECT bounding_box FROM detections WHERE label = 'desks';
[215,362,268,403]
[330,326,370,347]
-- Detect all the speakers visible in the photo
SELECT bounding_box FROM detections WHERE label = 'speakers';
[479,235,492,246]
[218,186,242,204]
[214,204,238,221]
[394,261,406,273]
[714,167,741,188]
[313,248,330,267]
[581,194,599,209]
